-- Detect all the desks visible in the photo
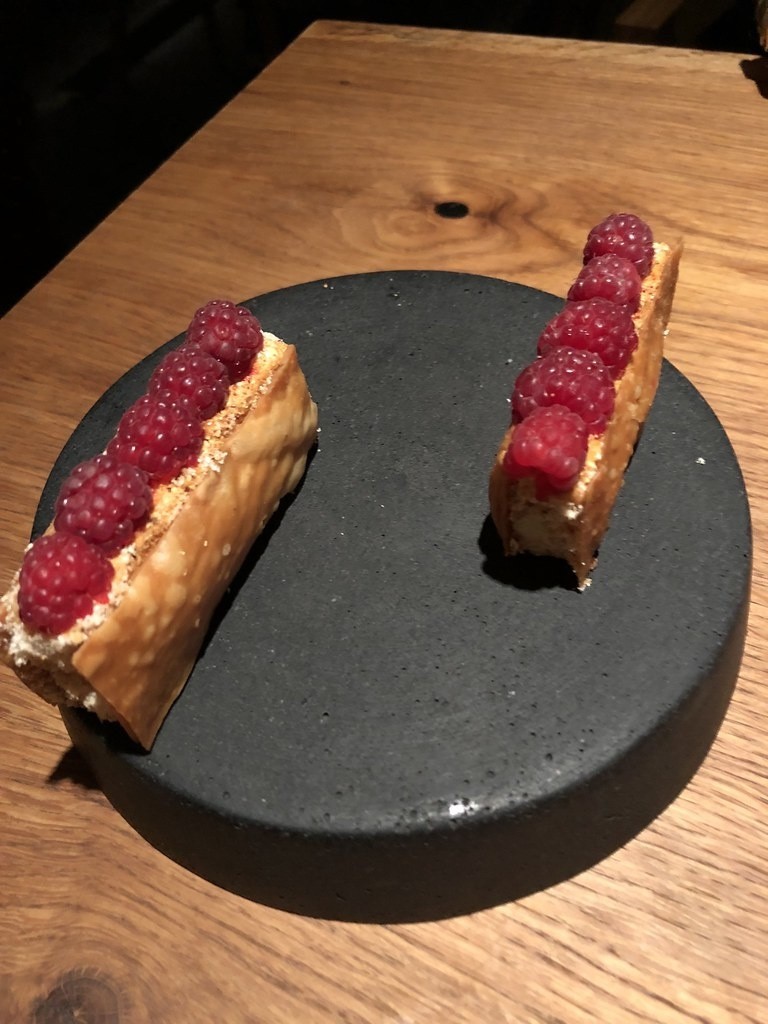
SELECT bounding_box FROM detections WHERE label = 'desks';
[0,20,768,1024]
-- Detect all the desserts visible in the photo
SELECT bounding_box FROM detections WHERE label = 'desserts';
[487,213,683,590]
[0,298,320,754]
